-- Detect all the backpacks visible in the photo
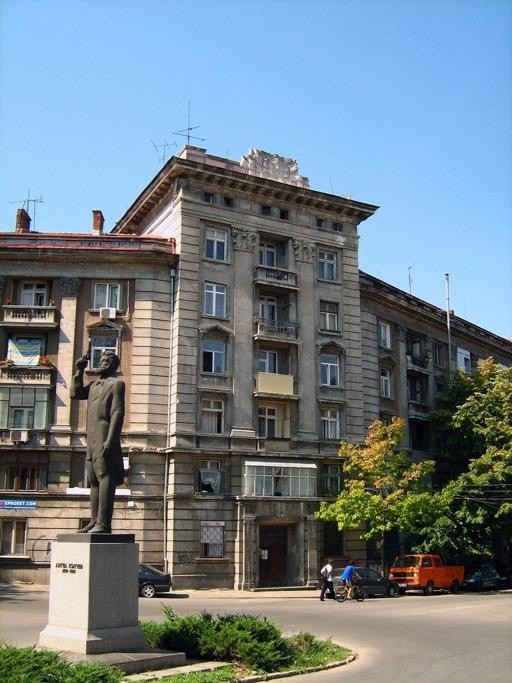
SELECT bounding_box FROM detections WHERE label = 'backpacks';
[320,566,332,584]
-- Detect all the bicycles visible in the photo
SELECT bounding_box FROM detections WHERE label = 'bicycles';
[334,577,366,603]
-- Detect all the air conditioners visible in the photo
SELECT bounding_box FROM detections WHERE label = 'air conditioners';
[9,430,29,445]
[99,307,116,319]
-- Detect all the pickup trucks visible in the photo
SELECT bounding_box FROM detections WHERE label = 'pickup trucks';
[389,553,467,596]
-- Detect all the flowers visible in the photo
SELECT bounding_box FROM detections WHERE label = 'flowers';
[36,353,55,367]
[0,358,15,367]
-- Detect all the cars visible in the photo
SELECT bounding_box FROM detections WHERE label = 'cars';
[137,561,172,600]
[323,566,401,600]
[461,561,512,592]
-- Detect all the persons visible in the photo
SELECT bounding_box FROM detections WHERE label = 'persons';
[340,562,362,599]
[70,351,125,533]
[320,560,339,601]
